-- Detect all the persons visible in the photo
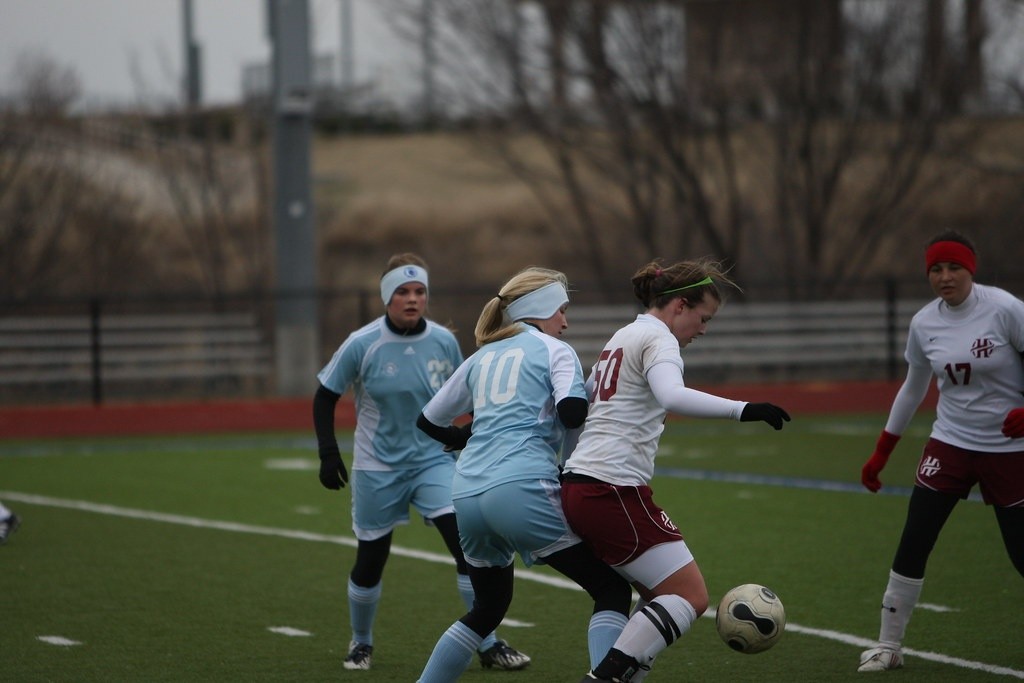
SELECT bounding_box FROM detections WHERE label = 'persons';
[311,252,534,672]
[563,258,793,682]
[412,266,638,683]
[859,231,1024,671]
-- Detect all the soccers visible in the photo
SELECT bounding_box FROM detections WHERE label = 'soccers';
[717,584,786,654]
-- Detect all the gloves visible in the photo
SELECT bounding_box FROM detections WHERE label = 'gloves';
[740,403,793,430]
[319,448,348,490]
[861,428,903,492]
[1002,408,1024,439]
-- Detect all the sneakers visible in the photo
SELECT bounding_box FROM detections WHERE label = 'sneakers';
[857,648,904,672]
[477,642,530,670]
[346,639,372,669]
